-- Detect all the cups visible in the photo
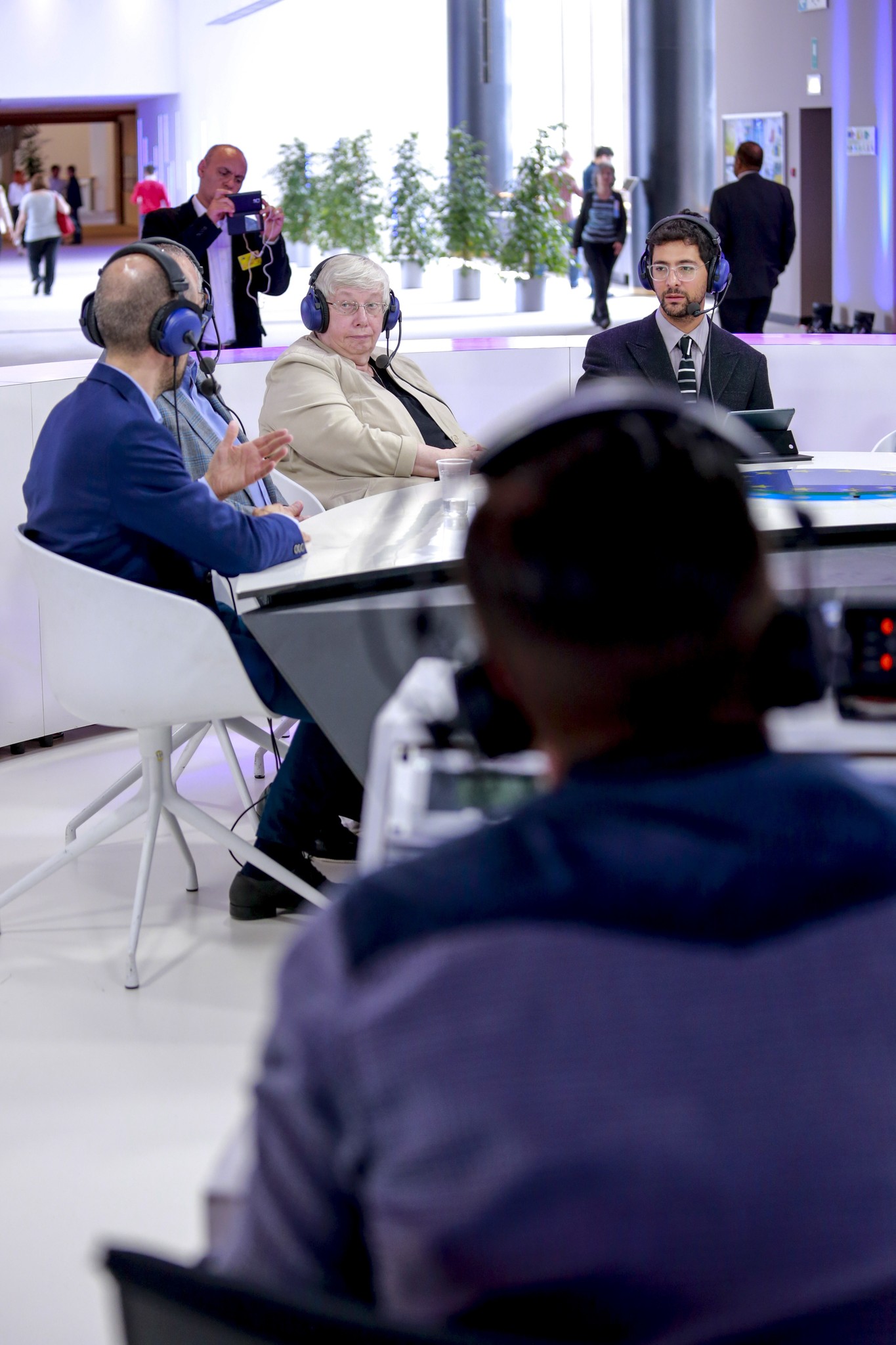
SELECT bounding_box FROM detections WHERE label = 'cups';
[438,458,473,515]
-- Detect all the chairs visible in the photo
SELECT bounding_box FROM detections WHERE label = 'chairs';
[251,455,327,780]
[17,517,337,993]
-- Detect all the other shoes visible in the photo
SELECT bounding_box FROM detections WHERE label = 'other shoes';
[591,305,610,329]
[33,277,51,296]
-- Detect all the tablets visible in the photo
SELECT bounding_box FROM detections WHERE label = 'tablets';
[721,407,814,464]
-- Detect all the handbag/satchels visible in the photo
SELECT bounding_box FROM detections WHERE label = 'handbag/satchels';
[55,193,75,235]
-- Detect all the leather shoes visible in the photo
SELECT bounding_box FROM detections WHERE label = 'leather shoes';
[229,859,354,920]
[255,782,359,860]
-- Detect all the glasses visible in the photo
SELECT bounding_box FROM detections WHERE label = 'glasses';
[326,300,389,316]
[647,260,710,282]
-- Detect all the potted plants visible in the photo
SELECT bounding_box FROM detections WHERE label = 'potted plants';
[431,125,506,303]
[266,127,384,268]
[496,121,581,312]
[383,128,441,290]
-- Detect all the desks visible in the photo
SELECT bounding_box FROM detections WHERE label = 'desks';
[237,447,896,800]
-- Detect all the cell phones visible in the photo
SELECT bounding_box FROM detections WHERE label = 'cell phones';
[224,191,262,216]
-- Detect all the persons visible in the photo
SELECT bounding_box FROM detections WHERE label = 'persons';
[11,172,72,296]
[572,209,775,421]
[139,144,293,352]
[129,164,172,241]
[65,165,83,245]
[48,163,68,195]
[133,235,366,863]
[0,183,16,252]
[570,160,627,329]
[8,168,28,256]
[534,150,585,290]
[582,145,616,299]
[706,139,797,333]
[20,253,366,922]
[209,389,896,1344]
[260,253,488,510]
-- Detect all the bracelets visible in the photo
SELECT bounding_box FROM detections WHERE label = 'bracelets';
[14,229,22,237]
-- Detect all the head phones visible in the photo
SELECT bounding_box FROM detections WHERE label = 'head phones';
[79,236,214,357]
[638,214,730,293]
[407,374,830,757]
[300,253,400,334]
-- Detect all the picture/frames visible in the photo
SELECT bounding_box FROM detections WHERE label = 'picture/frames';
[721,111,788,189]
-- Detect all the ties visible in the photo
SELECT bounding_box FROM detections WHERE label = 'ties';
[676,336,698,408]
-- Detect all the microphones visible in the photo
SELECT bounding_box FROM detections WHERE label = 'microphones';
[354,486,459,748]
[375,310,402,369]
[688,273,732,317]
[189,311,221,396]
